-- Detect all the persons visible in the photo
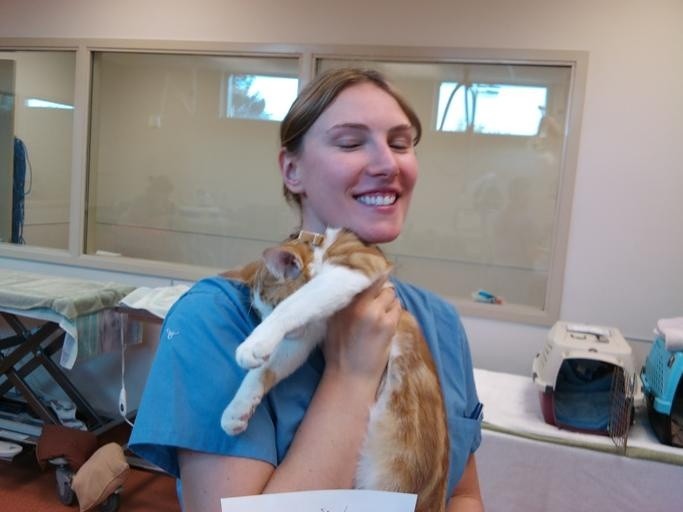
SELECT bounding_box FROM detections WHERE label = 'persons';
[124,67,487,512]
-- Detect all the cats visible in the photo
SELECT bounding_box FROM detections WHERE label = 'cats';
[218,224,450,512]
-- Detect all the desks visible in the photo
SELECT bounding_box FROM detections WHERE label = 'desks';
[472,365,683,512]
